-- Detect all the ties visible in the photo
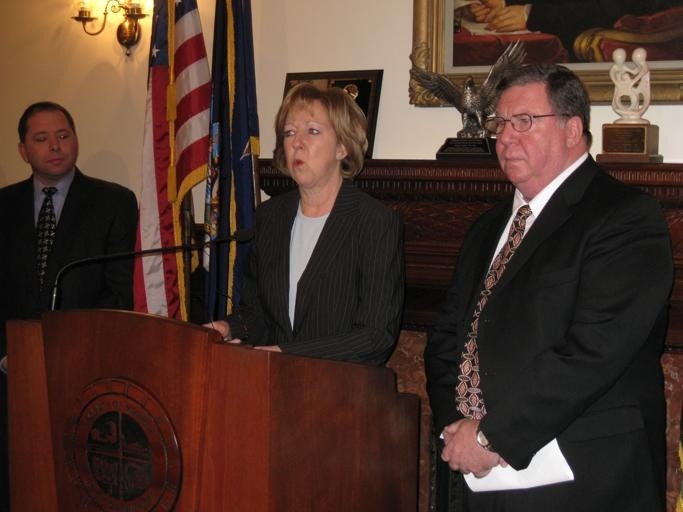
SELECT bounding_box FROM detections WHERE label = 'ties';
[454,206,531,421]
[33,187,57,294]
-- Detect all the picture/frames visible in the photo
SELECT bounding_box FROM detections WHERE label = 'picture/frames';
[279,67,384,160]
[403,0,683,112]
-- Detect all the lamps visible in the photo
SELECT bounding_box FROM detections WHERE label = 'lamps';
[71,0,150,61]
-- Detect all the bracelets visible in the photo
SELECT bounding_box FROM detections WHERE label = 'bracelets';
[473,424,500,454]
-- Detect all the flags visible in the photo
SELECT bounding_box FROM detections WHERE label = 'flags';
[200,0,264,323]
[131,0,211,314]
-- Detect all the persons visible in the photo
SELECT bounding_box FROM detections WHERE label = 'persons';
[198,82,408,369]
[0,101,142,419]
[420,60,672,512]
[464,0,681,53]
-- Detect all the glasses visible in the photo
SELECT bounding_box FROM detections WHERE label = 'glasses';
[484,112,565,132]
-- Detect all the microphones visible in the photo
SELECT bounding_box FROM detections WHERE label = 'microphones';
[51,228,255,310]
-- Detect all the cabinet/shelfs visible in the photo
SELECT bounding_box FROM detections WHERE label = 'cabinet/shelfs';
[251,152,683,511]
